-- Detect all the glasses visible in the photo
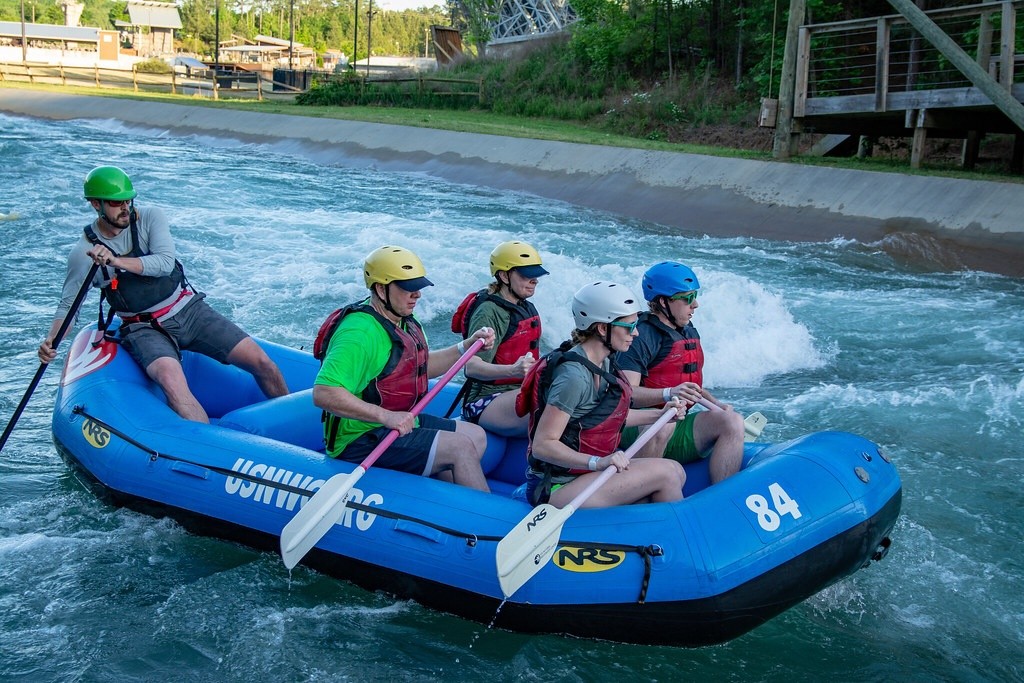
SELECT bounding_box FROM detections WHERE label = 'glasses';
[673,290,698,305]
[104,199,131,206]
[611,318,640,333]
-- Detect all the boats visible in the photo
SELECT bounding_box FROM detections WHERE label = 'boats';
[50,313,906,650]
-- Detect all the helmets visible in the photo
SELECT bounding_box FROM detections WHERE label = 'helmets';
[489,240,550,279]
[642,260,701,303]
[571,280,641,332]
[364,245,434,293]
[83,167,138,202]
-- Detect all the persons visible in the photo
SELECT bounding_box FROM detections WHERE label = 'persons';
[451,240,551,437]
[311,244,496,495]
[38,165,290,425]
[608,261,746,485]
[513,280,687,509]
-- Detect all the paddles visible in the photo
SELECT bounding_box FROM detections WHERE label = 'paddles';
[279,326,489,569]
[0,259,110,451]
[697,390,768,442]
[494,407,679,598]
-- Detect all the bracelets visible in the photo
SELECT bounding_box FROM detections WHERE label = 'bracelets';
[457,340,466,355]
[663,387,671,404]
[588,455,600,471]
